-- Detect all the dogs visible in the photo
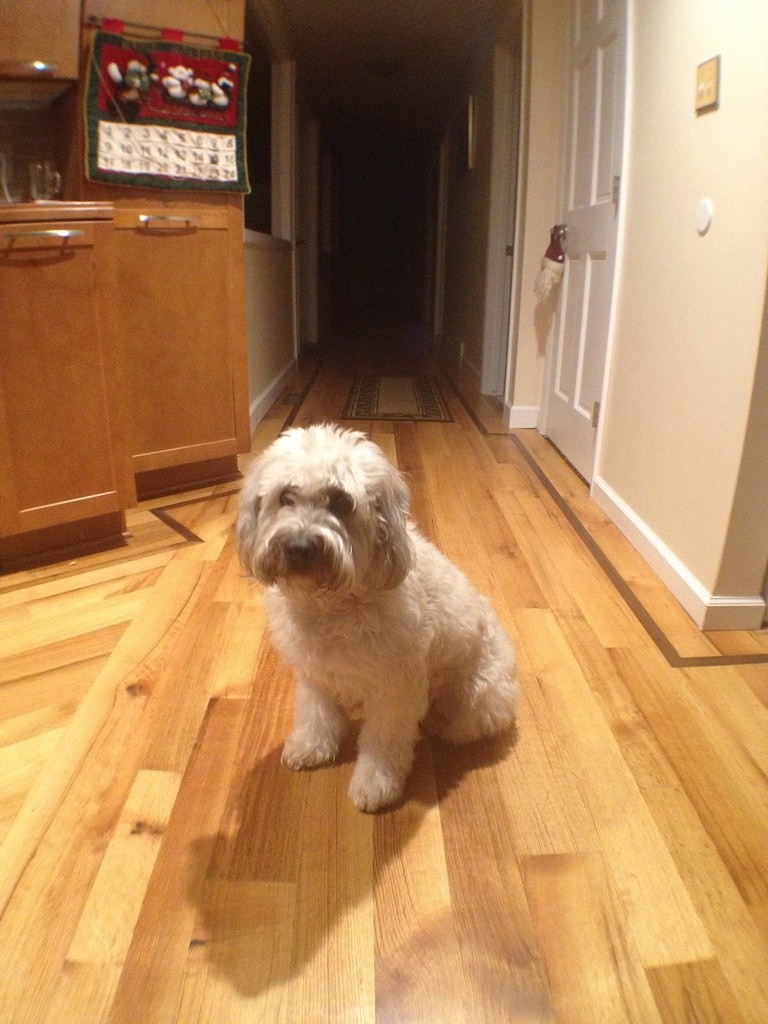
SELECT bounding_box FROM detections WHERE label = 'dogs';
[232,423,518,812]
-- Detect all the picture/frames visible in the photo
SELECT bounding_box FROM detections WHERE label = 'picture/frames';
[693,55,720,111]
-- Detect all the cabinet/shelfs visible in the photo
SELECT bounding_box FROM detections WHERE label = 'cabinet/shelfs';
[0,201,137,571]
[54,0,252,500]
[0,0,81,112]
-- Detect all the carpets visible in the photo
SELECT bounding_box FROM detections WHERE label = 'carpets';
[341,364,456,422]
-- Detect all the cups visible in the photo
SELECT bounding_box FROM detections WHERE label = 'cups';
[29,161,61,200]
